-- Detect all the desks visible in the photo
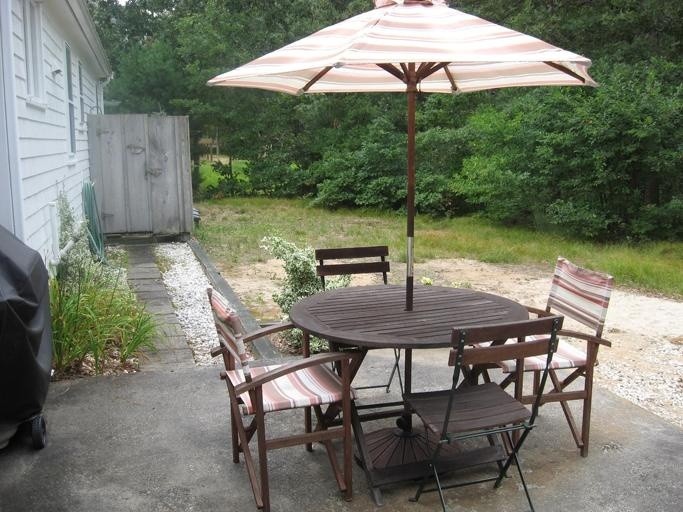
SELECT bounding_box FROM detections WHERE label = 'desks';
[287,284,532,478]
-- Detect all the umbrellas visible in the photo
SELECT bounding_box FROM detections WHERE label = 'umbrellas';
[206,0,600,311]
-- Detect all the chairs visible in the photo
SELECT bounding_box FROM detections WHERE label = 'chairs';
[309,245,405,426]
[473,256,615,457]
[402,315,564,509]
[206,282,359,511]
